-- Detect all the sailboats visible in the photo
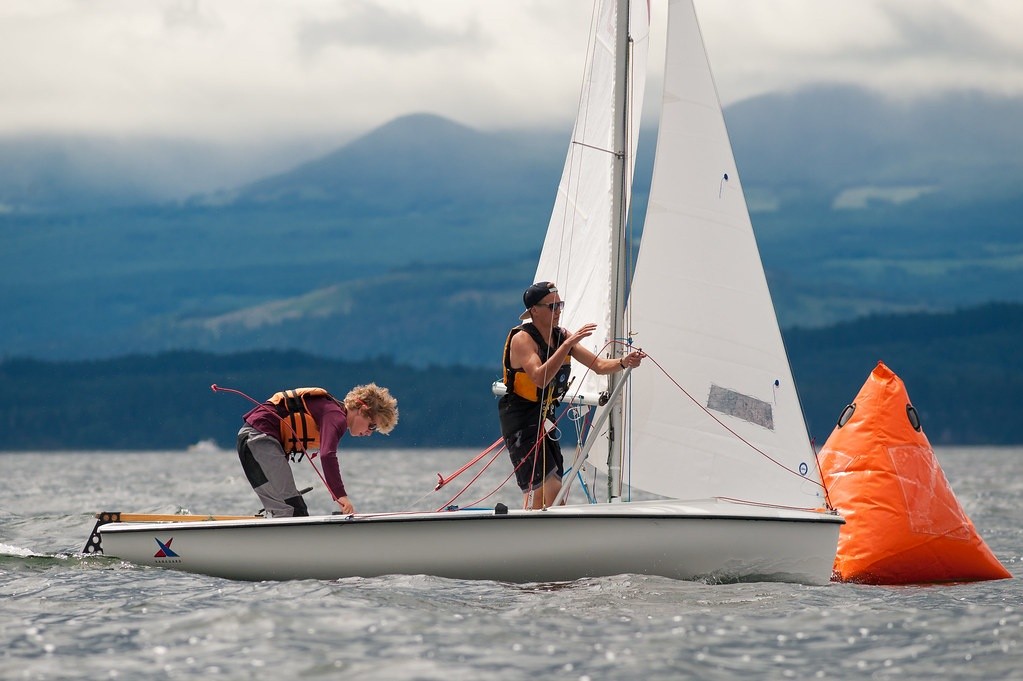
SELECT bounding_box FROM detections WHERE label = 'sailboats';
[81,0,845,589]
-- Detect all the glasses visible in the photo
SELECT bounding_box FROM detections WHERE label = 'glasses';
[358,400,377,430]
[535,301,564,312]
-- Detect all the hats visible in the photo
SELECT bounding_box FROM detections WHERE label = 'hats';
[519,282,558,319]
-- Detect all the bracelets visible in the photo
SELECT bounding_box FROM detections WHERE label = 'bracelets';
[619,358,625,369]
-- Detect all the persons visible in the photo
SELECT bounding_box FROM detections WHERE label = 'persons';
[235,382,400,518]
[498,282,646,510]
